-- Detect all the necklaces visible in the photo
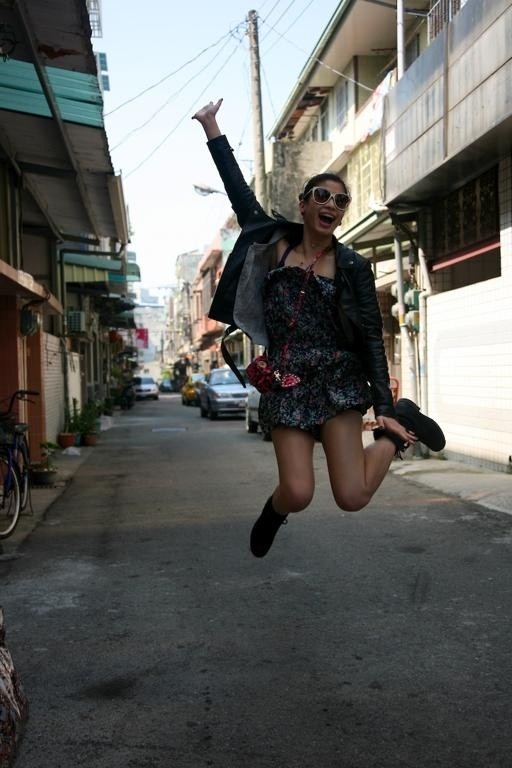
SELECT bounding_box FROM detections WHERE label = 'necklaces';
[302,227,332,250]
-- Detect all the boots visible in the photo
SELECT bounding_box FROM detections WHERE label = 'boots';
[248,492,290,559]
[369,395,447,461]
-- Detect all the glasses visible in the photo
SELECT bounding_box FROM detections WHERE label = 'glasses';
[299,185,352,213]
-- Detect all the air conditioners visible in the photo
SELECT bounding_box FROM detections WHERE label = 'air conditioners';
[68,311,86,334]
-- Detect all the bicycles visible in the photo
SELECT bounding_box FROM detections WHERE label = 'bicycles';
[0,389,39,539]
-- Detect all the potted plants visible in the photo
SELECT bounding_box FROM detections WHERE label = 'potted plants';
[31,441,59,486]
[57,395,105,447]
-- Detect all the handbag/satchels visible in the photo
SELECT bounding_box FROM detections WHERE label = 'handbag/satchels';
[242,353,302,396]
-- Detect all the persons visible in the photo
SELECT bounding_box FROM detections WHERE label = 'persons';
[190,97,446,560]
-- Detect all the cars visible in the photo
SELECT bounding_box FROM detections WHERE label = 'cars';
[132,373,160,401]
[180,362,273,442]
